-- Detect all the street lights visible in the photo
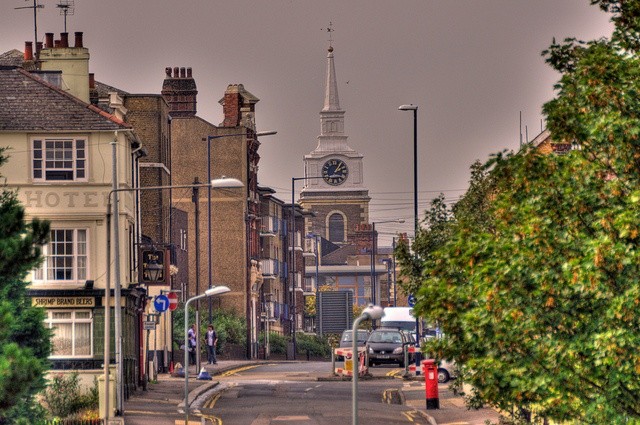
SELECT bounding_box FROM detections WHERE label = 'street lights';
[398,103,421,374]
[104,175,245,424]
[291,172,342,356]
[372,218,405,326]
[185,285,231,423]
[352,302,384,424]
[207,129,278,363]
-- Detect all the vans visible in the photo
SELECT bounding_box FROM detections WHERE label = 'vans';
[340,328,370,346]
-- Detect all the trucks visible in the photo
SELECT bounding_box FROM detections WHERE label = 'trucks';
[380,306,437,340]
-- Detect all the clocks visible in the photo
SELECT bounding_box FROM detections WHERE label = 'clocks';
[320,158,350,186]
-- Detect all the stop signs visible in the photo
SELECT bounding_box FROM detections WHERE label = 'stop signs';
[167,291,178,310]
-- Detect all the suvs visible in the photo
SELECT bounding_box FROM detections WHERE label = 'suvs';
[365,327,407,367]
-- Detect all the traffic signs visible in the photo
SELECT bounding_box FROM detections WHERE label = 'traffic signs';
[154,295,169,311]
[408,294,417,305]
[143,321,155,328]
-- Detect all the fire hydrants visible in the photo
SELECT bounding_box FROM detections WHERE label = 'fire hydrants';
[422,360,439,408]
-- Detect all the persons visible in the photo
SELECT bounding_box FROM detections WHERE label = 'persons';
[188,323,197,366]
[203,324,219,365]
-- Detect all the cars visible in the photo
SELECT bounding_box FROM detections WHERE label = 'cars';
[404,332,415,362]
[408,357,458,381]
